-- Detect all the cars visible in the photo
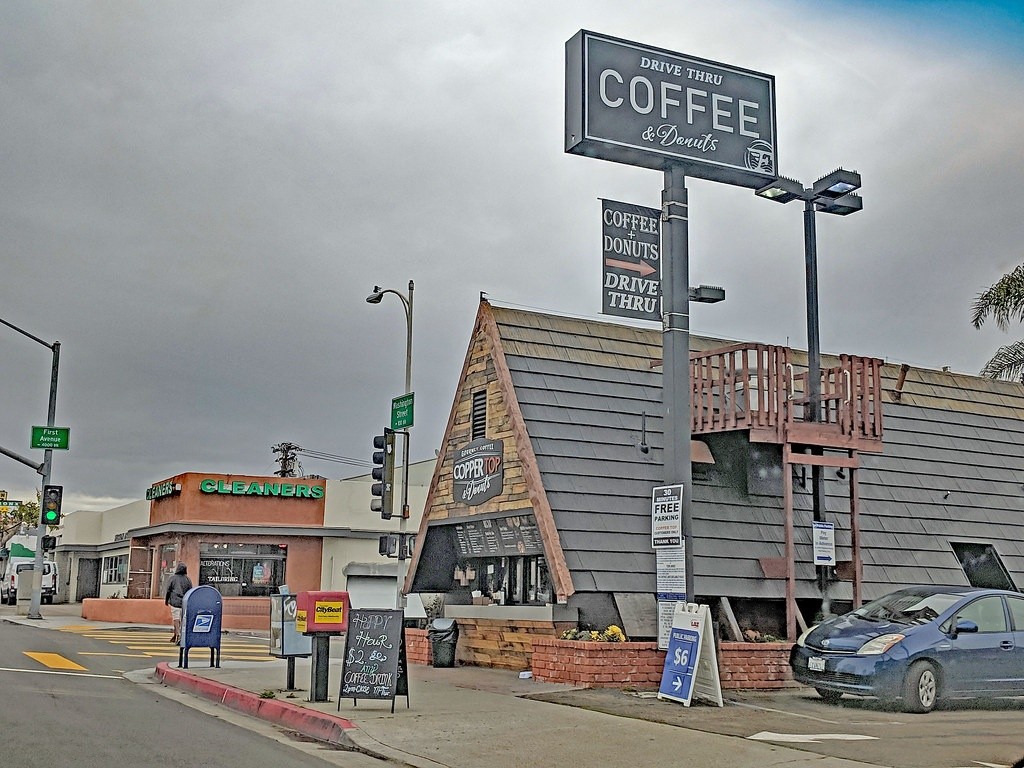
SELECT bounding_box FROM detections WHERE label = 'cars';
[789,586,1024,714]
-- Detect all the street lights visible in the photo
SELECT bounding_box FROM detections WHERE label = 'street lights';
[753,167,863,616]
[366,280,415,611]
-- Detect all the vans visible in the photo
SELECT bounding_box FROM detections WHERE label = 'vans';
[0,562,58,605]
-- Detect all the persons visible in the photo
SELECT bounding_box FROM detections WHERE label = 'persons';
[165,563,193,645]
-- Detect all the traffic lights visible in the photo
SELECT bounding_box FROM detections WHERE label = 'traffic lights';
[42,537,56,550]
[379,536,396,554]
[370,427,395,520]
[40,485,63,524]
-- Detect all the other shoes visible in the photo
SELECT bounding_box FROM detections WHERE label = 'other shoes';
[170,634,176,642]
[175,636,181,645]
